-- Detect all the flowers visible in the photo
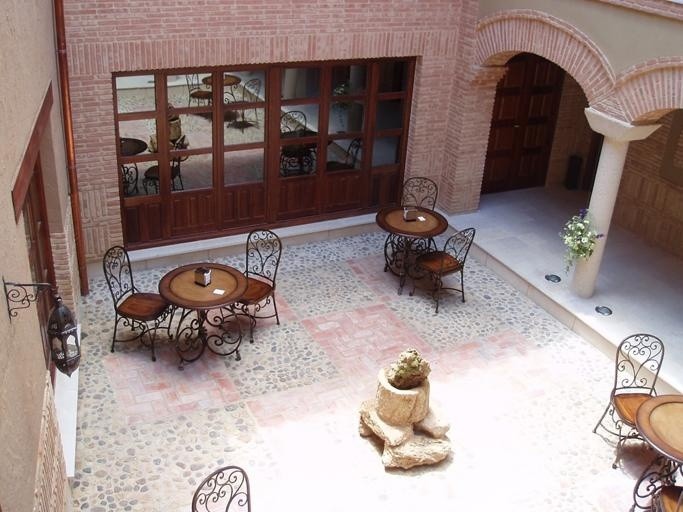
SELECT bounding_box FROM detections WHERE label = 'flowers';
[558,208,604,273]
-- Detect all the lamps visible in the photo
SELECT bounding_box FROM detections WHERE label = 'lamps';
[2,276,81,376]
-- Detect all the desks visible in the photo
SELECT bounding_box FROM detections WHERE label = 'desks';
[202,75,240,119]
[279,131,332,178]
[613,395,683,512]
[376,206,448,296]
[159,263,248,370]
[120,138,147,196]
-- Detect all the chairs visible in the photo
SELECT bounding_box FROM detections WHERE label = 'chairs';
[186,73,212,118]
[142,135,185,195]
[228,79,261,133]
[192,466,250,512]
[220,228,282,344]
[410,228,475,313]
[592,334,664,469]
[327,138,360,171]
[280,111,306,136]
[390,177,438,266]
[652,486,683,512]
[103,246,173,361]
